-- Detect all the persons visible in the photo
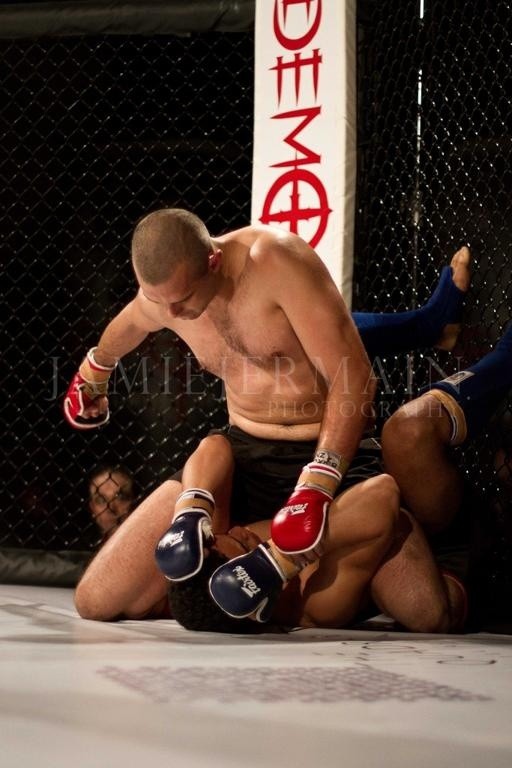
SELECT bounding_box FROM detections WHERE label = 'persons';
[154,244,510,631]
[86,465,136,536]
[65,208,472,632]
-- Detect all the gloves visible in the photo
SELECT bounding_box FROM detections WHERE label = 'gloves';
[64,346,119,430]
[209,539,306,623]
[155,488,216,583]
[271,462,342,555]
